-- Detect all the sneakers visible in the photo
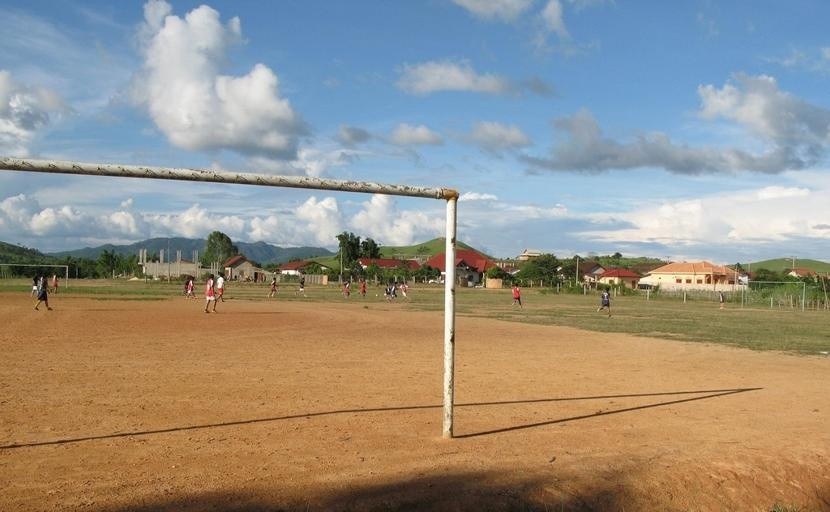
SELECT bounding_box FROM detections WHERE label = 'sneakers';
[204,309,216,313]
[33,306,52,310]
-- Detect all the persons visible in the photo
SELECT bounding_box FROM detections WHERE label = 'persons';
[596,288,613,317]
[267,276,278,297]
[718,290,726,310]
[30,276,40,297]
[216,272,225,303]
[31,271,54,312]
[48,271,59,294]
[510,282,523,309]
[202,272,217,313]
[182,279,190,296]
[341,278,412,302]
[294,277,308,298]
[185,275,198,299]
